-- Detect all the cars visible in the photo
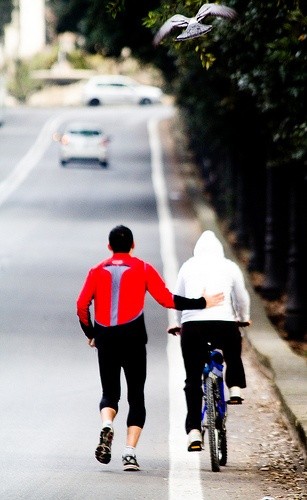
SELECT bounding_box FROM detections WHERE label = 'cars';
[66,75,165,109]
[56,128,111,168]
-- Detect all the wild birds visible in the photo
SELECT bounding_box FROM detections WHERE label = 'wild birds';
[154,3,236,45]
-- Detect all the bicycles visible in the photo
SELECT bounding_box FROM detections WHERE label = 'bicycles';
[189,345,242,472]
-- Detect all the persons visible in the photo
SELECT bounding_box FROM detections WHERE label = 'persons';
[167,230,250,451]
[77,225,224,471]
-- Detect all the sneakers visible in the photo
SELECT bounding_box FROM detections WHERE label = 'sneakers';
[230,386,244,401]
[122,453,141,470]
[94,424,114,464]
[187,429,202,451]
